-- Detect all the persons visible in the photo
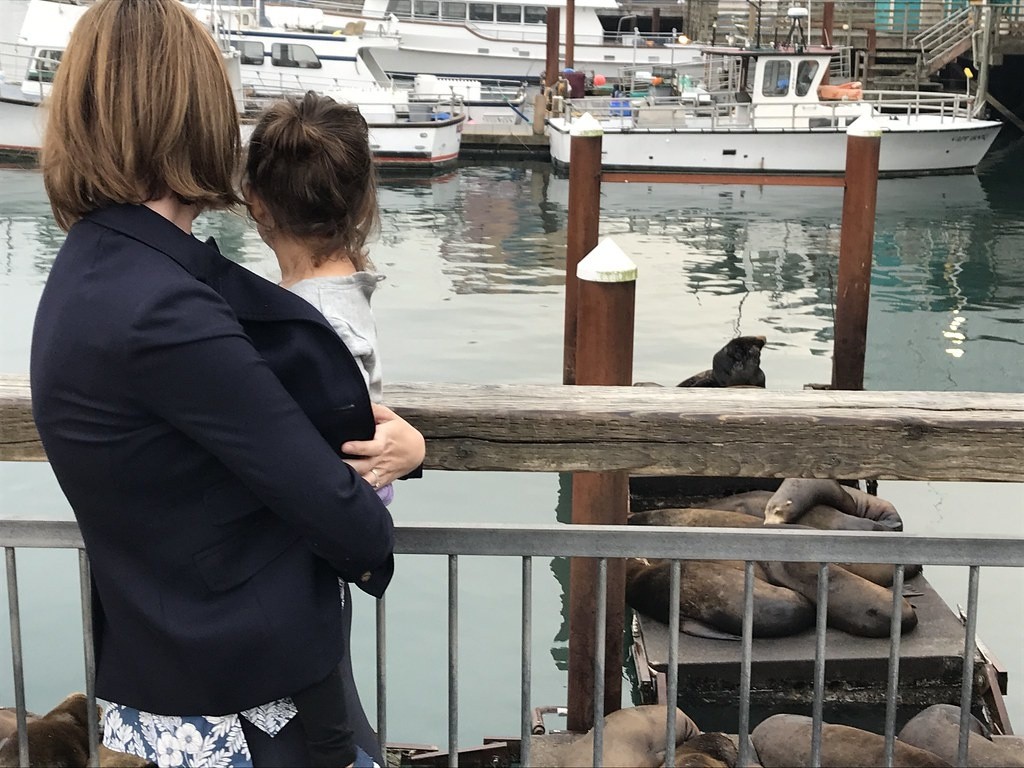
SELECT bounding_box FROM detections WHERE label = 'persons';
[30,0,427,768]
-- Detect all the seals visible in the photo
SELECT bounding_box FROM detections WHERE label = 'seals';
[0,333,1023,767]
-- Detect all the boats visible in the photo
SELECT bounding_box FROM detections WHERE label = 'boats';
[546,161,989,300]
[0,0,743,93]
[0,25,464,171]
[545,6,1004,175]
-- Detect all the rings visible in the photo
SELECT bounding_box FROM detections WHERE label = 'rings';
[370,469,380,488]
